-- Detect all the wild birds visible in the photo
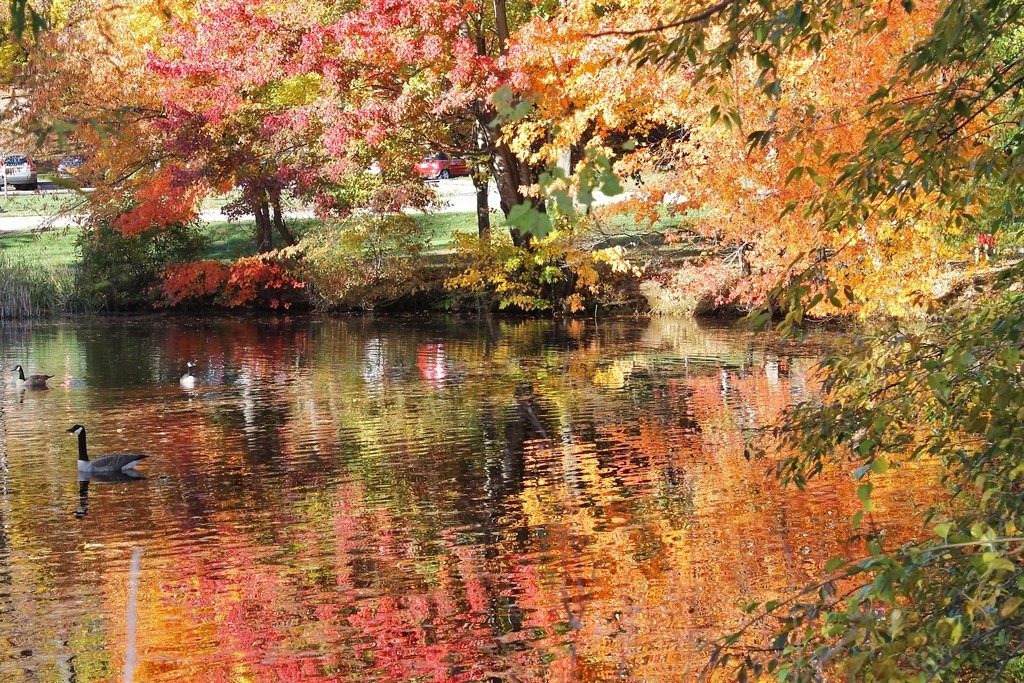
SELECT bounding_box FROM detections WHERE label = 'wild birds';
[10,365,54,386]
[179,361,197,383]
[66,424,149,472]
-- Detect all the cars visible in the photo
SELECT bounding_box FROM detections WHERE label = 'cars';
[415,152,469,178]
[58,154,104,187]
[1,153,38,189]
